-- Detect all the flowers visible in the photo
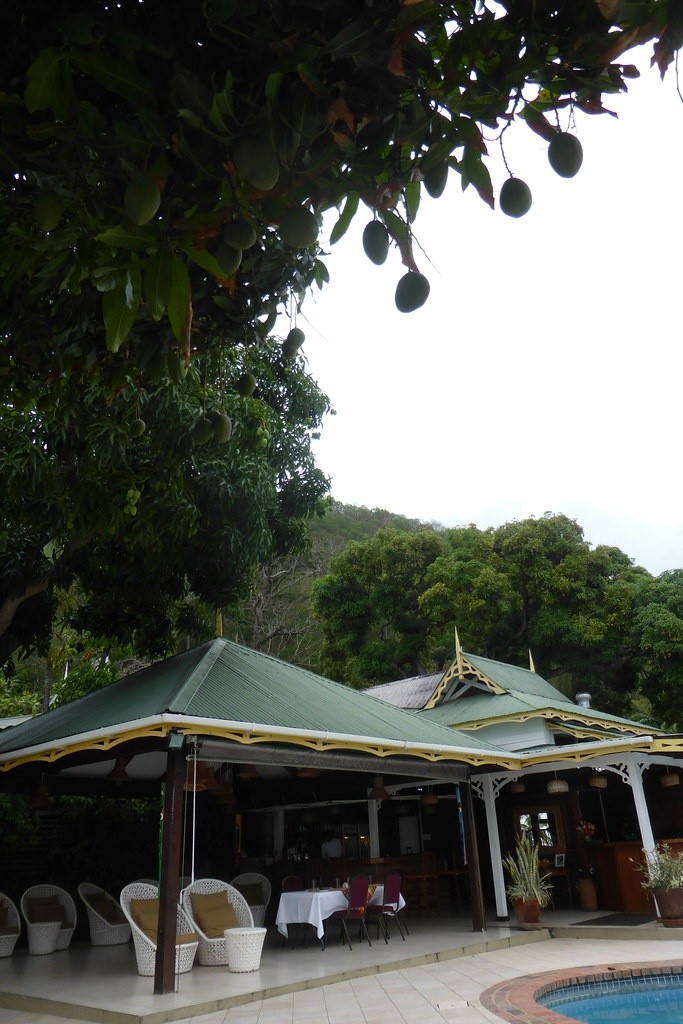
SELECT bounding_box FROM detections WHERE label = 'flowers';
[574,814,596,841]
[575,864,601,885]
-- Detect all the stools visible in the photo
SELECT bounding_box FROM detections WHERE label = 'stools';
[224,927,267,973]
[28,921,62,955]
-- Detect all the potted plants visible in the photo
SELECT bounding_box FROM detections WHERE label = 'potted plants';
[502,828,555,923]
[629,842,683,928]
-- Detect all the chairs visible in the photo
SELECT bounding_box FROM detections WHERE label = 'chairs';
[362,869,407,944]
[120,882,200,977]
[20,885,77,951]
[182,878,255,967]
[229,872,272,926]
[77,881,132,946]
[408,871,443,917]
[0,891,21,959]
[324,873,373,951]
[383,869,410,935]
[281,875,306,949]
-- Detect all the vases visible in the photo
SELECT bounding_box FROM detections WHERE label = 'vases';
[577,878,600,912]
[584,835,593,843]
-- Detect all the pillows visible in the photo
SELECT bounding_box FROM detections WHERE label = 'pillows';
[85,891,106,909]
[138,912,158,941]
[0,906,9,927]
[233,881,262,905]
[95,900,118,921]
[197,903,239,938]
[27,894,59,921]
[31,903,65,928]
[190,890,228,925]
[130,898,159,924]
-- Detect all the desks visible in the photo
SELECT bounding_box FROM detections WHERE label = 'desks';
[275,883,407,951]
[407,871,472,912]
[539,866,574,912]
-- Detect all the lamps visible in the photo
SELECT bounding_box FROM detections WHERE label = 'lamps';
[422,784,440,816]
[105,756,134,785]
[184,759,239,806]
[660,765,681,789]
[587,770,608,789]
[508,782,527,794]
[367,773,391,801]
[546,770,569,795]
[27,772,50,811]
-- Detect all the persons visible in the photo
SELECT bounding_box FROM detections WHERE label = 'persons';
[321,830,343,864]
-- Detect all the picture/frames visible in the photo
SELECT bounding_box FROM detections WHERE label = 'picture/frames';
[555,853,565,867]
